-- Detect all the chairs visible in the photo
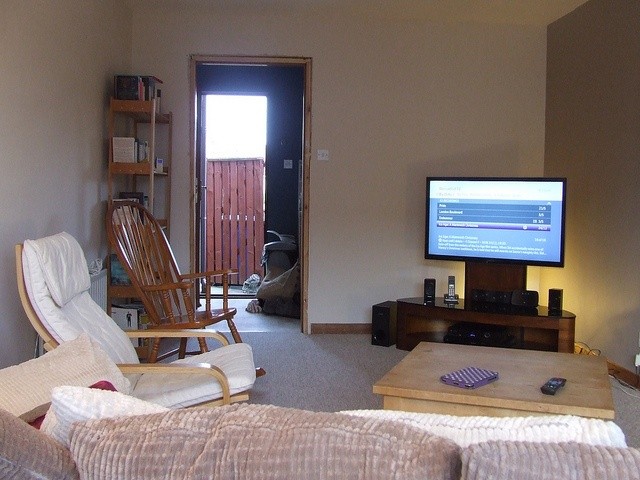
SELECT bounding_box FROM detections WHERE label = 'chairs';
[105,200,267,377]
[14,235,256,411]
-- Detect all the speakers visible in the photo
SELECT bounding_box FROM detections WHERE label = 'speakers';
[423,278,436,307]
[548,288,563,317]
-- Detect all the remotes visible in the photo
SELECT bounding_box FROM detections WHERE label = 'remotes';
[541,376,567,396]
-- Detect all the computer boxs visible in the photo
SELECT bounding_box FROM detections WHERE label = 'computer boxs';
[372,300,396,347]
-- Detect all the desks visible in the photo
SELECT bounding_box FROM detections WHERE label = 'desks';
[395,262,576,352]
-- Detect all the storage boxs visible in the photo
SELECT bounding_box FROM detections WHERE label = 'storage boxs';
[117,76,155,101]
[112,302,152,347]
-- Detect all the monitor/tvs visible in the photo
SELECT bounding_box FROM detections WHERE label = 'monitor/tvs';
[424,176,567,268]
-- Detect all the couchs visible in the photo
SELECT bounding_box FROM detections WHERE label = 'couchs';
[0,332,637,480]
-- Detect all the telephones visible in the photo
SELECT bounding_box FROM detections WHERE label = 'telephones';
[444,276,459,304]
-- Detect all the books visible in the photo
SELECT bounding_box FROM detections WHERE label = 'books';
[110,252,131,284]
[111,302,147,347]
[112,136,147,164]
[112,191,148,225]
[114,74,160,114]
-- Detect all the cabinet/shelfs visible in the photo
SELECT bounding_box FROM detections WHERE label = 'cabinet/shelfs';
[103,96,172,359]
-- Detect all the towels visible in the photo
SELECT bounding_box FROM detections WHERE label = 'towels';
[439,366,498,389]
